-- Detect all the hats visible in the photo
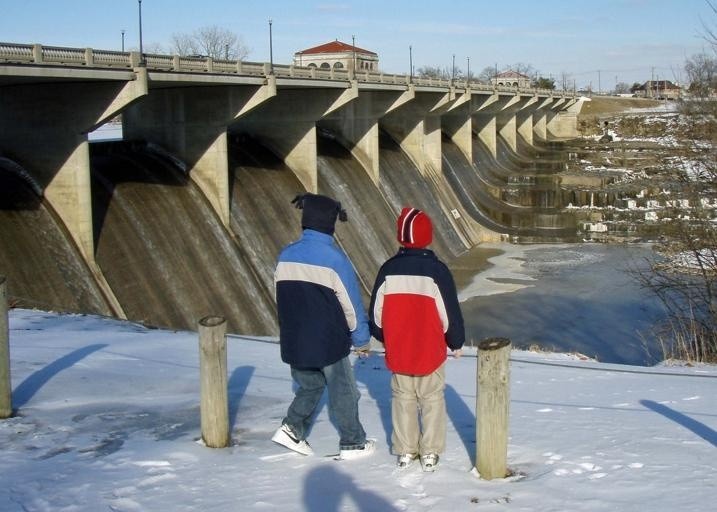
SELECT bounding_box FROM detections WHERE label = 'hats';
[291,192,348,235]
[396,207,434,249]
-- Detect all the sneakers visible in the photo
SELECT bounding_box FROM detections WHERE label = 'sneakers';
[339,439,377,461]
[397,451,441,473]
[271,423,316,456]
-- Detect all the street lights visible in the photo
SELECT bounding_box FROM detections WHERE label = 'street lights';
[351,33,357,80]
[408,45,414,84]
[451,53,471,87]
[119,29,127,53]
[225,43,229,61]
[267,15,275,75]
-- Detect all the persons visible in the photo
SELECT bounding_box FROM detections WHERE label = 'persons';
[269,191,377,462]
[367,206,466,472]
